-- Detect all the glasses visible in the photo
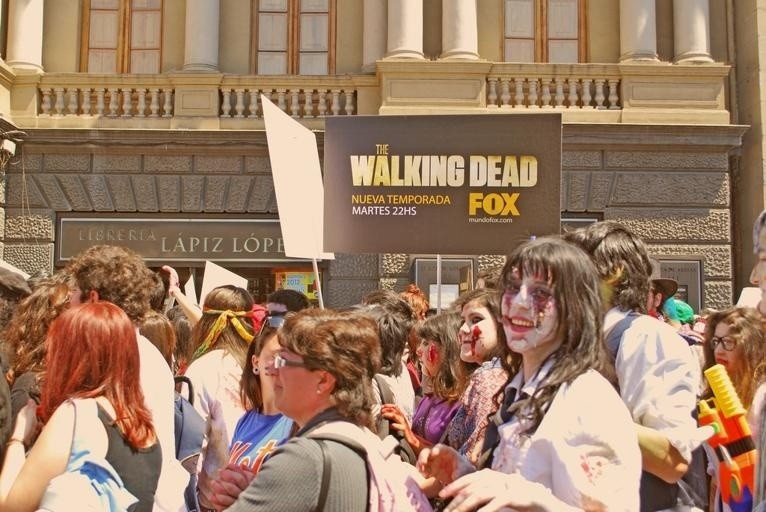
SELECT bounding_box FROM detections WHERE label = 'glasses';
[270,354,308,370]
[709,336,739,352]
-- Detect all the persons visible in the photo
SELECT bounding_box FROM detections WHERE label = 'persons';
[0,209,766,512]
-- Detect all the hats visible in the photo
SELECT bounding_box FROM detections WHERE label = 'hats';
[646,257,679,301]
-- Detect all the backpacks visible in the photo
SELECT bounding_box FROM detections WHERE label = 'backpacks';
[36,394,140,512]
[300,419,435,512]
[174,393,208,512]
